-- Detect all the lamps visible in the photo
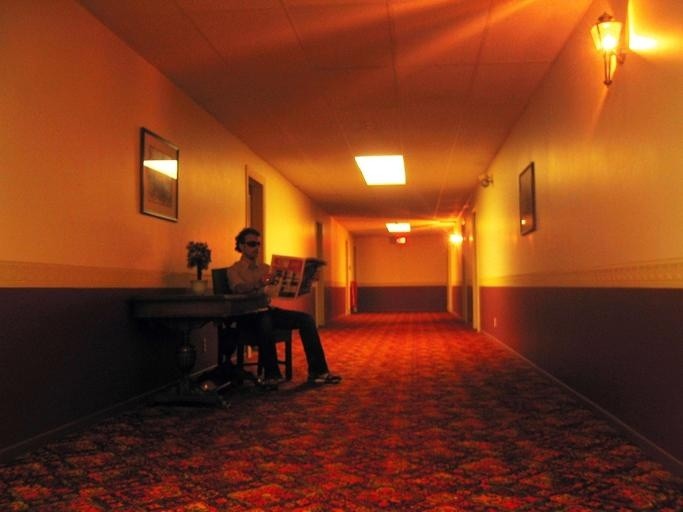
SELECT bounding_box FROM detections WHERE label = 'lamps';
[477,174,492,187]
[589,12,625,87]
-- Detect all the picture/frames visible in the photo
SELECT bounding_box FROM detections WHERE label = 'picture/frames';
[518,161,536,235]
[140,126,180,223]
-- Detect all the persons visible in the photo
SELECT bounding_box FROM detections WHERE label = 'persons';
[226,227,342,391]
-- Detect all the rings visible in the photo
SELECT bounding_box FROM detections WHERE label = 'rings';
[266,278,270,282]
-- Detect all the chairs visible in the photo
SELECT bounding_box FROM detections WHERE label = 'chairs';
[210,267,293,380]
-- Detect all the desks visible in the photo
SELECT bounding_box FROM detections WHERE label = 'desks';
[130,293,269,404]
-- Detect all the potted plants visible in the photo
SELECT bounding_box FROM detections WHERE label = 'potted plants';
[186,239,212,293]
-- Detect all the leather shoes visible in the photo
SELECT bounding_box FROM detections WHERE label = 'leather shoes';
[263,376,278,389]
[305,373,341,384]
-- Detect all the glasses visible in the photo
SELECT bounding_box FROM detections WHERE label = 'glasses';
[242,239,261,248]
